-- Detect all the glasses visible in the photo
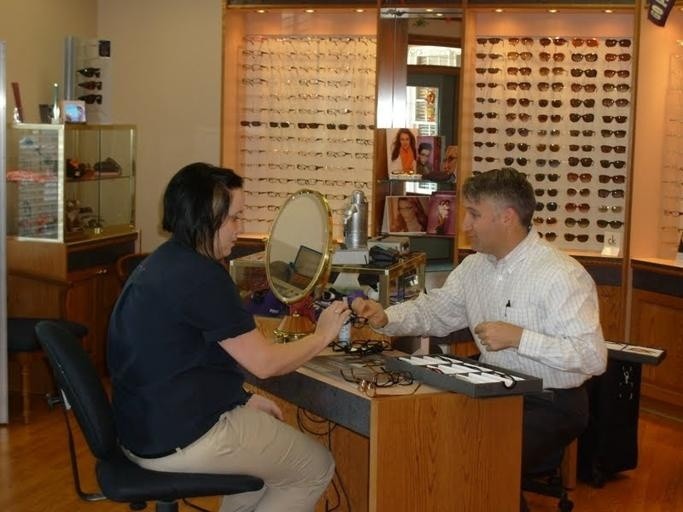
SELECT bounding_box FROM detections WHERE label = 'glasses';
[339,367,422,398]
[420,153,429,157]
[343,313,368,329]
[448,156,458,161]
[472,36,631,243]
[76,68,102,104]
[332,339,392,359]
[492,367,515,381]
[239,34,377,224]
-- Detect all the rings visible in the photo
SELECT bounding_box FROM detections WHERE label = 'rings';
[332,308,341,316]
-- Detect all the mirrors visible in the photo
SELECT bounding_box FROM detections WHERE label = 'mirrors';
[264,188,336,339]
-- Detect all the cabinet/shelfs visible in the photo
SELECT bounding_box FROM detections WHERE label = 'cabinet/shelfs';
[230,250,426,309]
[7,123,140,400]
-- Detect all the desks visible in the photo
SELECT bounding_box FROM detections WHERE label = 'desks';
[7,317,89,424]
[241,338,554,512]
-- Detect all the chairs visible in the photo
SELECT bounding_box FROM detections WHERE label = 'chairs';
[35,320,263,512]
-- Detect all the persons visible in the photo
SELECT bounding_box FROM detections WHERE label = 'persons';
[351,168,609,459]
[108,163,351,512]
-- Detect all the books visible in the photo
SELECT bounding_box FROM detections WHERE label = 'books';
[382,127,457,237]
